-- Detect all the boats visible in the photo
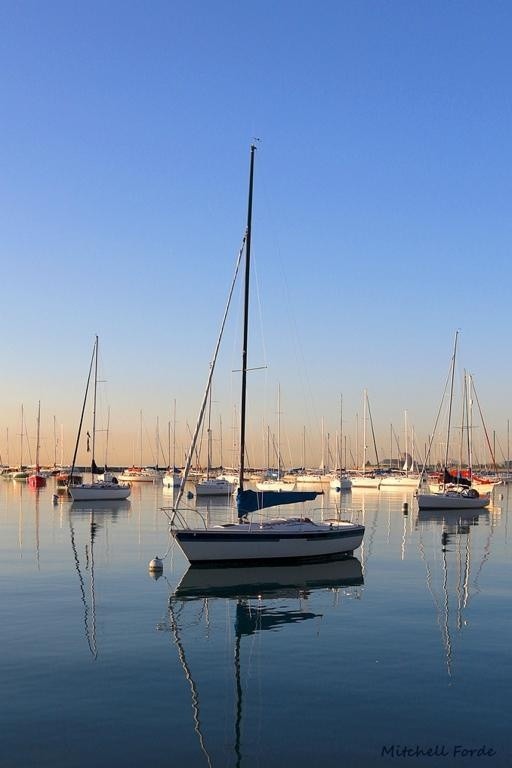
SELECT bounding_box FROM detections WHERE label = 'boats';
[169,518,365,566]
